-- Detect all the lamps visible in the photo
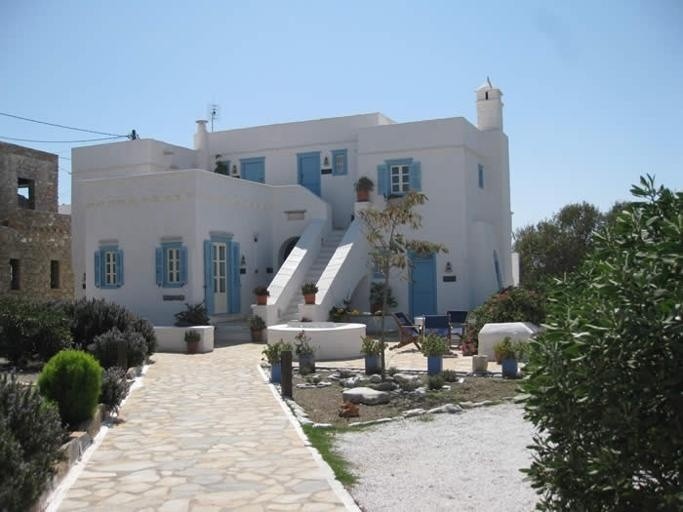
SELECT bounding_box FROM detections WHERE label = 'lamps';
[389,310,468,352]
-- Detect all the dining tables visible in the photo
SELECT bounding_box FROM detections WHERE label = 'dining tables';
[493,335,524,378]
[262,331,287,383]
[253,286,269,305]
[353,176,373,202]
[184,329,200,353]
[358,332,388,375]
[293,330,318,371]
[250,315,266,342]
[370,283,397,314]
[420,332,447,374]
[302,282,318,304]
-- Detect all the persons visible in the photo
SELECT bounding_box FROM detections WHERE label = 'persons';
[125,127,139,140]
